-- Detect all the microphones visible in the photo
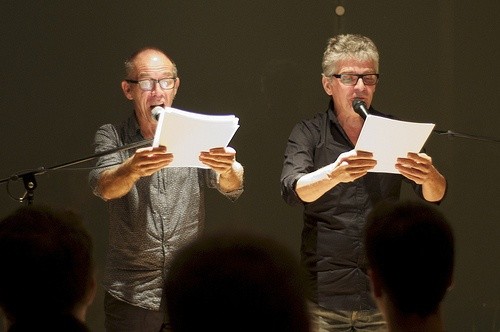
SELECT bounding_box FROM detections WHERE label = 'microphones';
[352,99,369,120]
[152,106,163,121]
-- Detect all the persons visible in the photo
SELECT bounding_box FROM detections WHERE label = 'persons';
[279,33,450,331]
[0,208,98,332]
[362,199,457,332]
[158,226,320,332]
[86,46,246,332]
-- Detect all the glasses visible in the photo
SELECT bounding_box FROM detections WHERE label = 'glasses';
[124,77,177,91]
[326,74,379,86]
